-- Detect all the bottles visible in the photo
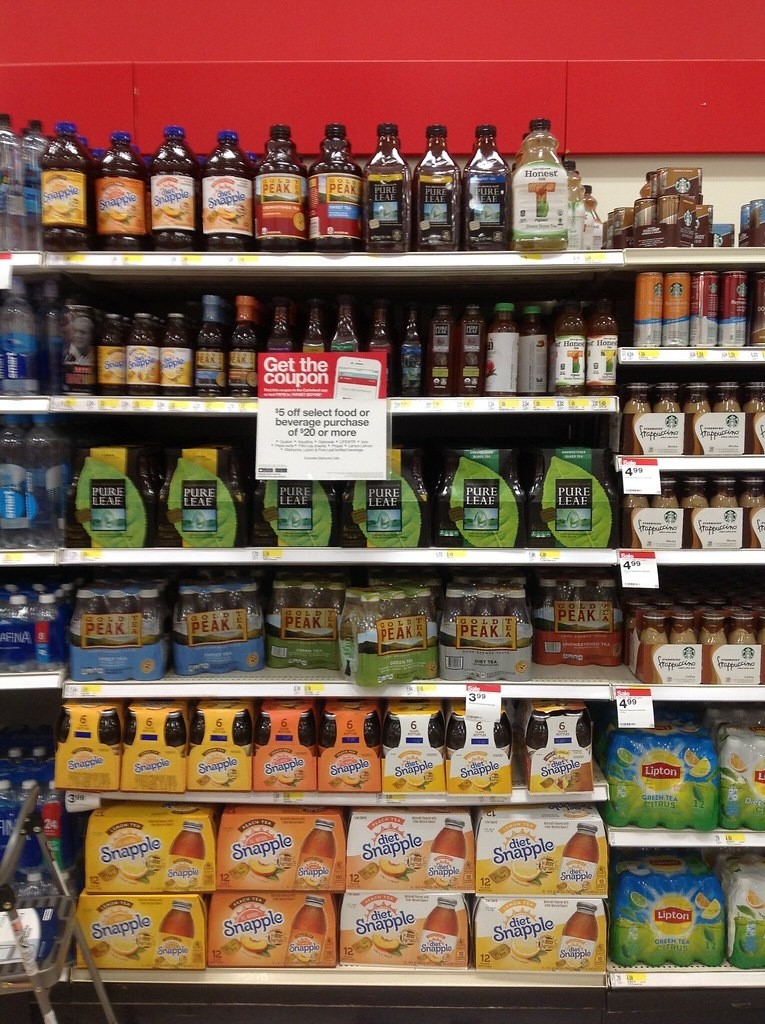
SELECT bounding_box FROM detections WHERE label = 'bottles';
[154,901,195,969]
[415,896,458,966]
[99,710,121,754]
[0,115,598,251]
[555,823,602,895]
[620,379,764,684]
[286,894,327,966]
[1,412,91,549]
[423,819,465,890]
[554,901,599,971]
[295,819,337,890]
[125,709,589,749]
[163,820,205,890]
[0,731,84,907]
[0,568,616,675]
[54,710,72,747]
[0,276,617,398]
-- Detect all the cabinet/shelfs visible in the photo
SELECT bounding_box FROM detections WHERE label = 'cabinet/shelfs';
[42,250,626,988]
[0,252,73,982]
[605,246,764,989]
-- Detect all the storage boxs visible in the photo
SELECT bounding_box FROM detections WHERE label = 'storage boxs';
[54,697,607,973]
